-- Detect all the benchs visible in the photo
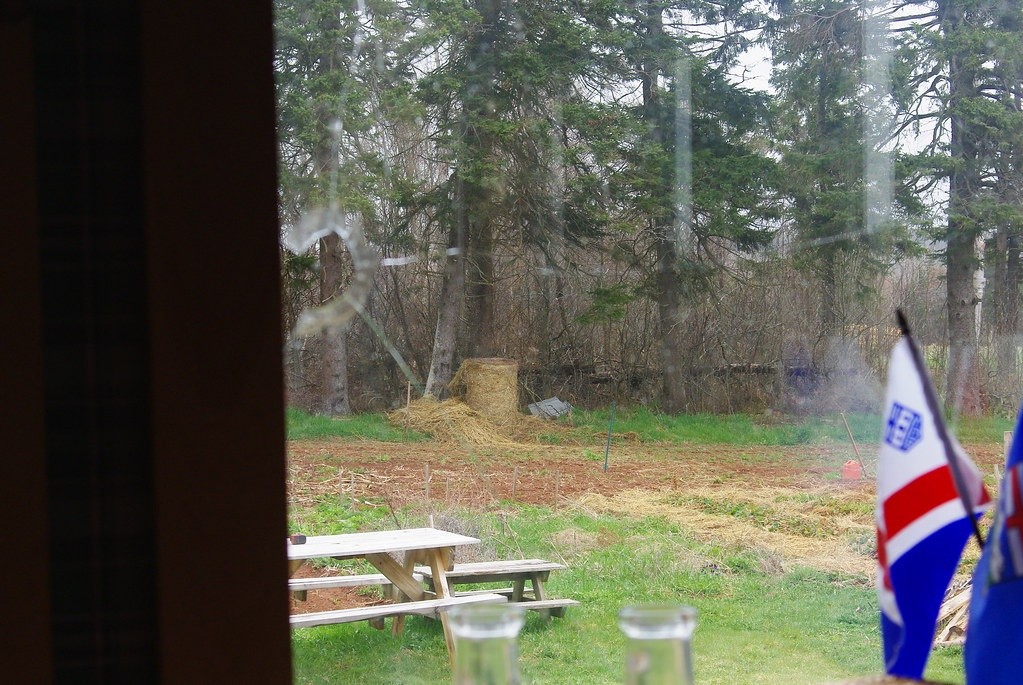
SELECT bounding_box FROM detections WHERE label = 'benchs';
[455,586,535,597]
[509,599,581,610]
[290,594,508,631]
[288,572,424,593]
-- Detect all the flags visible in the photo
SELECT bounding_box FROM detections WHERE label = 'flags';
[877,333,1023,685]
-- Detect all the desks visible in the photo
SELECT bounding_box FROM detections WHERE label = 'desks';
[287,528,481,685]
[414,559,567,622]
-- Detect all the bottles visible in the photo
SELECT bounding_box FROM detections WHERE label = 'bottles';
[619,603,697,685]
[436,602,527,685]
